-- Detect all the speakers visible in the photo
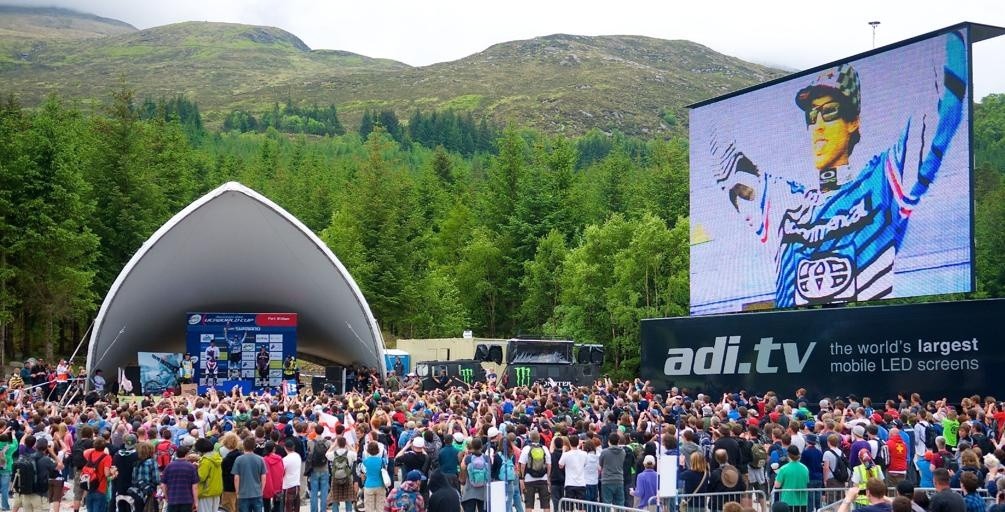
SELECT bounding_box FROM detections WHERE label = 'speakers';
[325,366,342,379]
[323,380,342,395]
[128,382,142,396]
[123,366,140,382]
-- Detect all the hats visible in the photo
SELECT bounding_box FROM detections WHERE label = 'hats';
[802,432,819,445]
[642,454,656,466]
[452,431,465,443]
[406,469,427,482]
[853,425,865,436]
[867,413,904,428]
[123,432,139,449]
[720,464,740,488]
[485,426,502,439]
[794,63,866,115]
[412,436,426,448]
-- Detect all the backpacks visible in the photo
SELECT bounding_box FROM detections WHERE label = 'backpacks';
[10,454,45,498]
[918,422,938,450]
[938,452,959,473]
[748,438,768,470]
[496,451,519,482]
[871,437,892,471]
[824,448,850,482]
[466,455,490,489]
[309,438,328,467]
[77,450,109,494]
[524,441,547,479]
[331,450,352,486]
[389,485,421,512]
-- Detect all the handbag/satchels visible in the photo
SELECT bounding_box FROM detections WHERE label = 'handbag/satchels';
[678,498,688,512]
[381,469,392,489]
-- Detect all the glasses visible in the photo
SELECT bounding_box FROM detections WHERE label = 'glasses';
[803,98,845,127]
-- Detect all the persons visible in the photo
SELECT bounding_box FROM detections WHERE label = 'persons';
[703,27,966,308]
[0,328,1005,512]
